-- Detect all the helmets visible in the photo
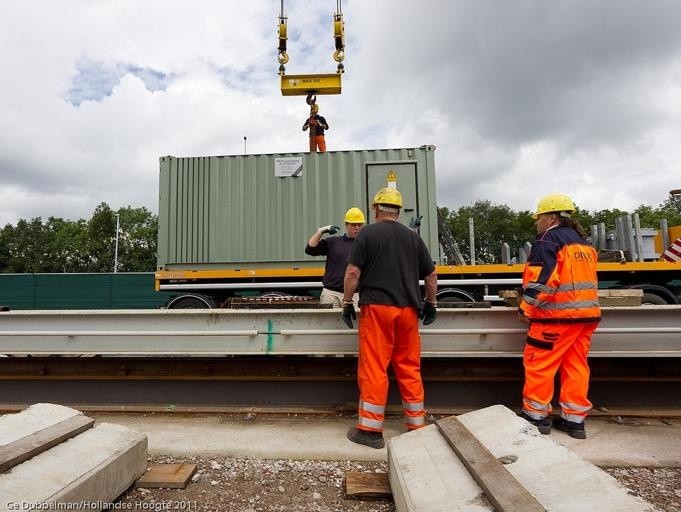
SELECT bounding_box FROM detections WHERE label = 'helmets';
[312,104,319,110]
[531,194,576,220]
[370,187,403,209]
[344,207,366,223]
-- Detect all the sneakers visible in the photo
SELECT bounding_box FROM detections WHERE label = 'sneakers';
[347,426,385,449]
[519,412,551,434]
[552,417,586,439]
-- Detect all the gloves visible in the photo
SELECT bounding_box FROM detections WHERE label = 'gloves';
[318,225,340,235]
[518,300,532,323]
[409,216,423,229]
[420,297,438,325]
[315,120,321,126]
[342,299,356,329]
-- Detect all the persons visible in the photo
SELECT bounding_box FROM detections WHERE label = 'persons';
[301,104,330,152]
[514,193,605,440]
[305,205,424,308]
[341,187,438,448]
